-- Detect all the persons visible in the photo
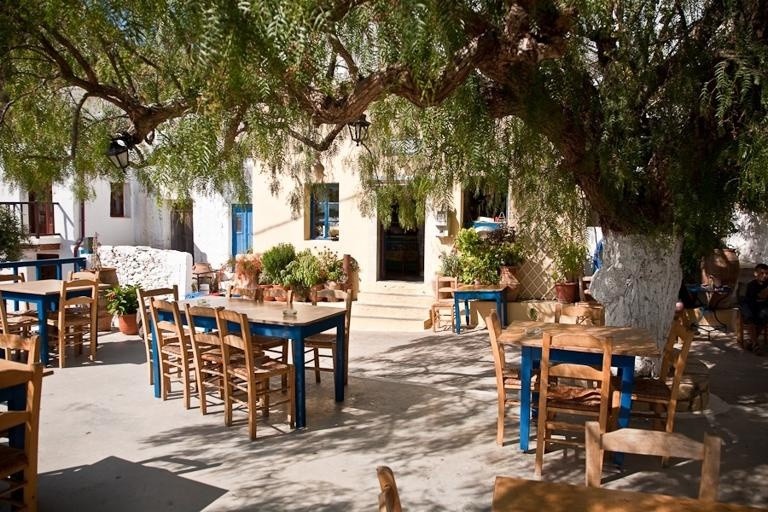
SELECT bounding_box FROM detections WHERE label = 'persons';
[744,263,768,355]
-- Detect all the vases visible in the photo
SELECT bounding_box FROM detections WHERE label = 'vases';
[697,248,740,307]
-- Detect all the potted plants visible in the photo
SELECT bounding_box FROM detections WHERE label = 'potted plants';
[106,284,144,336]
[281,261,307,302]
[297,255,324,301]
[551,233,590,303]
[321,244,342,301]
[258,270,274,300]
[262,243,295,302]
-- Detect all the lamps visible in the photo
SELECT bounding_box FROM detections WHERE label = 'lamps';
[347,113,372,144]
[106,135,131,175]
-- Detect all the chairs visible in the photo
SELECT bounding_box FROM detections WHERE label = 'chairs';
[630,319,695,463]
[213,284,264,339]
[0,334,43,365]
[67,270,100,356]
[0,362,42,512]
[293,286,353,386]
[251,289,294,392]
[150,296,218,411]
[136,284,200,384]
[216,307,295,442]
[431,275,458,332]
[0,293,38,360]
[375,465,402,512]
[184,304,265,414]
[555,303,605,326]
[583,420,723,500]
[1,273,34,359]
[48,279,98,369]
[535,332,614,475]
[486,308,560,447]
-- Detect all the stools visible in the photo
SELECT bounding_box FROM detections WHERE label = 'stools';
[736,310,768,344]
